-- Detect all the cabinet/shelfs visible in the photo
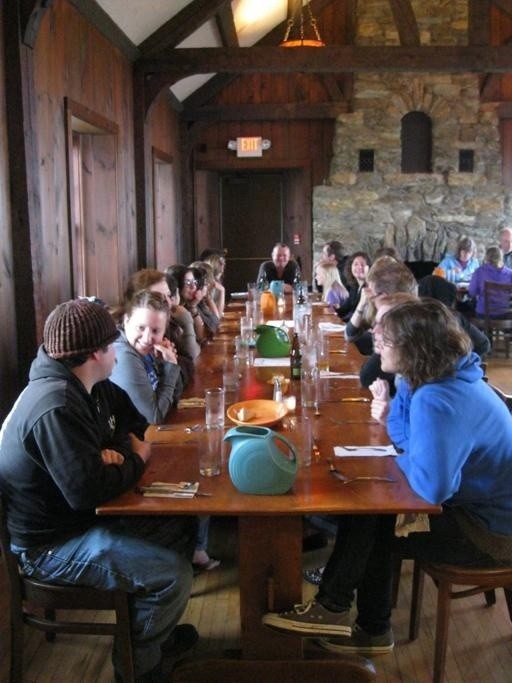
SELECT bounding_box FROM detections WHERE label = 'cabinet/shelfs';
[293,266,303,292]
[289,332,303,381]
[258,271,268,294]
[296,286,307,304]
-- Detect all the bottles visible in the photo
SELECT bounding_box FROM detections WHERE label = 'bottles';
[43,300,119,359]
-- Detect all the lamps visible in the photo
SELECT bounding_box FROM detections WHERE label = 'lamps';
[409,375,512,682]
[471,280,512,360]
[0,513,142,683]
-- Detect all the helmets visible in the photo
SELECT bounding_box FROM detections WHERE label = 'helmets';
[266,321,295,328]
[227,398,289,428]
[253,357,290,367]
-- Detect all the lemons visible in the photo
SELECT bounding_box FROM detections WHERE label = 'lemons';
[222,425,298,495]
[270,281,284,300]
[253,325,291,358]
[435,267,444,276]
[260,292,275,315]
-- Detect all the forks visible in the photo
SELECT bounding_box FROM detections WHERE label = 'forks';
[143,481,196,489]
[329,416,380,426]
[157,424,202,433]
[340,444,387,452]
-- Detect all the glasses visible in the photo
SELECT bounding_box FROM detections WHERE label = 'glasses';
[280,0,323,48]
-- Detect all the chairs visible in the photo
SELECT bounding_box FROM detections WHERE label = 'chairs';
[263,600,352,637]
[317,623,395,654]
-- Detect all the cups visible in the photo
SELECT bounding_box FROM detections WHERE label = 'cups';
[184,280,198,287]
[382,333,399,348]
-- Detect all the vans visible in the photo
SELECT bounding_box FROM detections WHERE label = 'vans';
[191,556,221,577]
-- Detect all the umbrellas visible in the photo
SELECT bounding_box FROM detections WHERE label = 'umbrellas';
[161,623,197,656]
[114,669,167,683]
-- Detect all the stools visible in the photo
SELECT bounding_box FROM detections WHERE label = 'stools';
[12,548,44,563]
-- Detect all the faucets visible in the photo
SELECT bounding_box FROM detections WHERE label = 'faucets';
[303,568,323,584]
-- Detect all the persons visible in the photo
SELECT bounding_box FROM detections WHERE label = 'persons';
[261,298,512,654]
[0,297,200,683]
[432,236,479,312]
[122,268,172,310]
[321,240,345,265]
[163,248,228,360]
[337,251,372,322]
[255,241,302,285]
[108,290,222,578]
[479,226,512,269]
[315,260,349,310]
[358,293,421,397]
[464,246,512,320]
[344,255,458,342]
[366,262,492,357]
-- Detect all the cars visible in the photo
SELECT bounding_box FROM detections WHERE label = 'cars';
[303,345,316,366]
[299,280,308,295]
[287,416,311,467]
[447,270,456,283]
[222,359,236,393]
[300,366,318,408]
[235,335,249,358]
[247,283,256,301]
[240,317,252,344]
[245,301,257,328]
[316,339,330,374]
[233,354,248,388]
[200,431,220,478]
[205,387,224,429]
[316,361,329,402]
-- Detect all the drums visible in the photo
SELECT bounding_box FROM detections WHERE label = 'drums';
[142,488,214,497]
[323,397,371,402]
[342,476,396,484]
